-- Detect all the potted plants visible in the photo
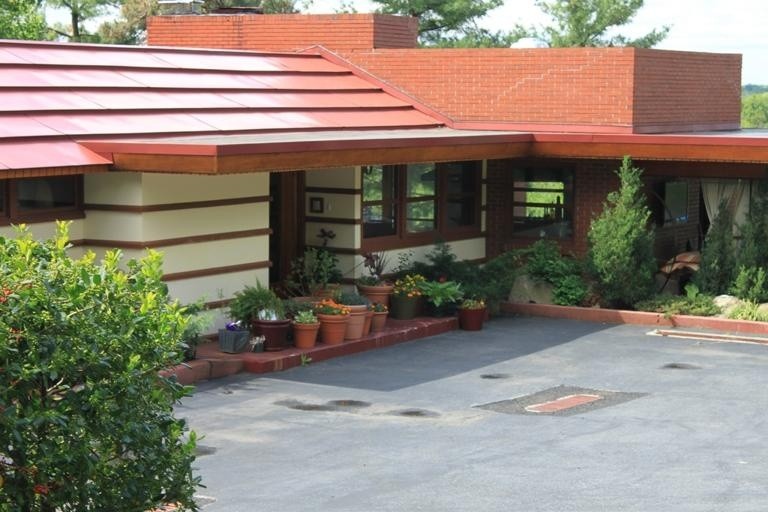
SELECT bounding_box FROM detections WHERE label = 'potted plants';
[310,197,324,213]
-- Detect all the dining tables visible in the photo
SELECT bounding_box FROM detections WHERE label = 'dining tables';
[218,228,487,353]
